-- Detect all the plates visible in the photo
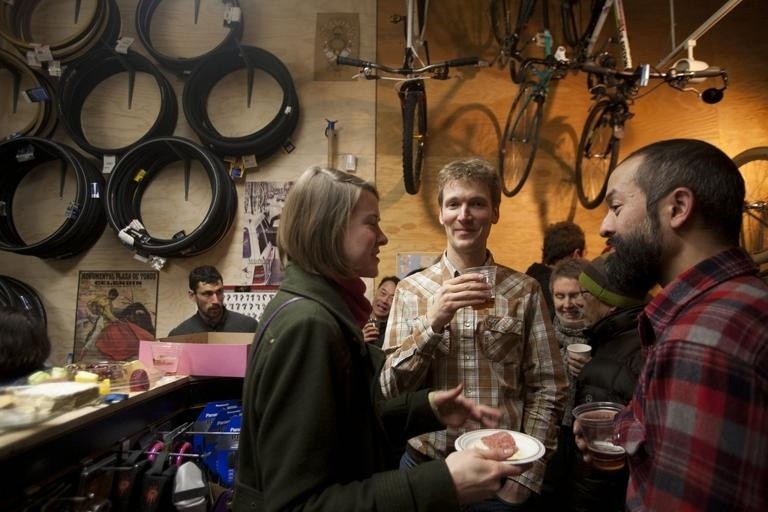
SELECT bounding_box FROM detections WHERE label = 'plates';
[455,427,546,466]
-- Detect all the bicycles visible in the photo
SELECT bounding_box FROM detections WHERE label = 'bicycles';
[729,147,768,285]
[488,0,605,198]
[560,0,730,209]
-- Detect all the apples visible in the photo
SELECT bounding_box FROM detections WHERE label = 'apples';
[129,369,149,392]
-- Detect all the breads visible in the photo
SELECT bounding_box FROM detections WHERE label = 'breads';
[480,431,519,457]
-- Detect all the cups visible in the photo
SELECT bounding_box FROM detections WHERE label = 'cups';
[367,317,385,344]
[566,344,594,366]
[570,401,631,470]
[463,265,497,309]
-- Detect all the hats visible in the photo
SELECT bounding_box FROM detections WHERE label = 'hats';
[579,252,658,310]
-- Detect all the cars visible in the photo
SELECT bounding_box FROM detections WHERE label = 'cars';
[336,0,477,195]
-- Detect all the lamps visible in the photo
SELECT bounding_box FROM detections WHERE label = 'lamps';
[655,0,741,82]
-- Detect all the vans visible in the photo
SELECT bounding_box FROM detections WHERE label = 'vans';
[241,212,281,286]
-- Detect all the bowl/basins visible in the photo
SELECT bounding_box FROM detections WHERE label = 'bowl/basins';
[65,360,132,385]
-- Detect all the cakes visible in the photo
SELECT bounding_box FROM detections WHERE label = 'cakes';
[15,381,99,413]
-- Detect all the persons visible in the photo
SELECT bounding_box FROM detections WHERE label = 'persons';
[524,139,767,512]
[362,276,401,349]
[379,158,571,512]
[167,264,258,337]
[231,164,534,512]
